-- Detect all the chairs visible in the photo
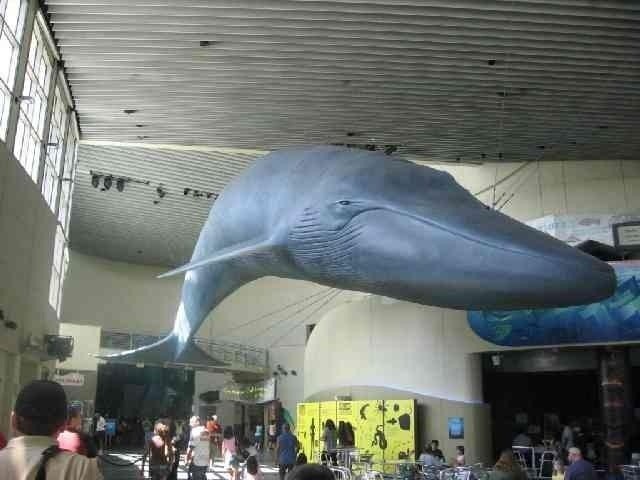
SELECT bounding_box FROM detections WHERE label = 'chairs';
[317,440,640,480]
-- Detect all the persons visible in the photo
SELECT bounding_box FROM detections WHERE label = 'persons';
[491,415,631,478]
[0,379,354,480]
[419,440,465,474]
